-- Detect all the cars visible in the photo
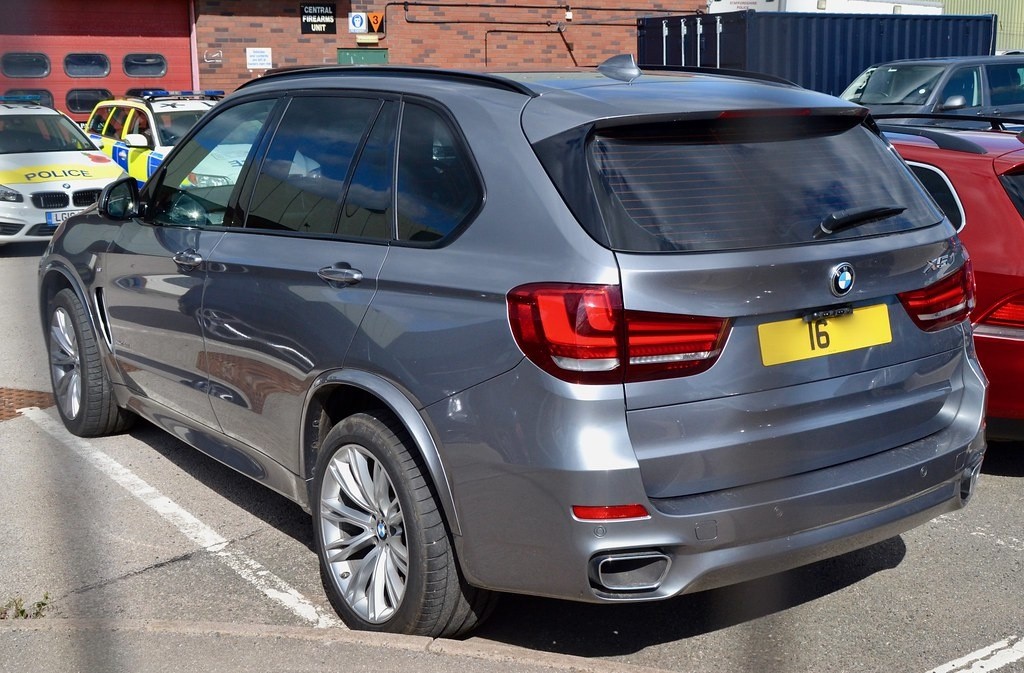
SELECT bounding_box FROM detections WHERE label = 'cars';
[858,54,1024,130]
[0,95,129,247]
[77,90,321,225]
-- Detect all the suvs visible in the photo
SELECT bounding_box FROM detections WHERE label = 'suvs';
[870,114,1024,444]
[39,53,991,640]
